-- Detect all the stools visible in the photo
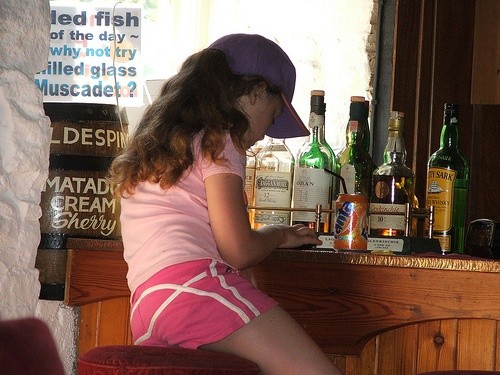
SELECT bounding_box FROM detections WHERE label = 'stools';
[74,337,260,375]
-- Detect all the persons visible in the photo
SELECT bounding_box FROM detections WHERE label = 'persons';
[110,33,347,375]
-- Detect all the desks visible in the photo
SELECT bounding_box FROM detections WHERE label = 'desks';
[63,227,500,375]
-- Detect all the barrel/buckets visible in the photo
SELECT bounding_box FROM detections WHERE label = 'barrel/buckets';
[34,102,130,301]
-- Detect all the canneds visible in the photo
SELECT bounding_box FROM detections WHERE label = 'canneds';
[334,193,368,252]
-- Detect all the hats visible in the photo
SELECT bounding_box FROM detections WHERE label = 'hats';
[209,33,311,138]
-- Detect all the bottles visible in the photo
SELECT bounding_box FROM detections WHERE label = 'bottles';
[368,111,415,255]
[423,102,470,254]
[327,96,375,236]
[243,147,256,229]
[290,90,335,235]
[252,138,295,230]
